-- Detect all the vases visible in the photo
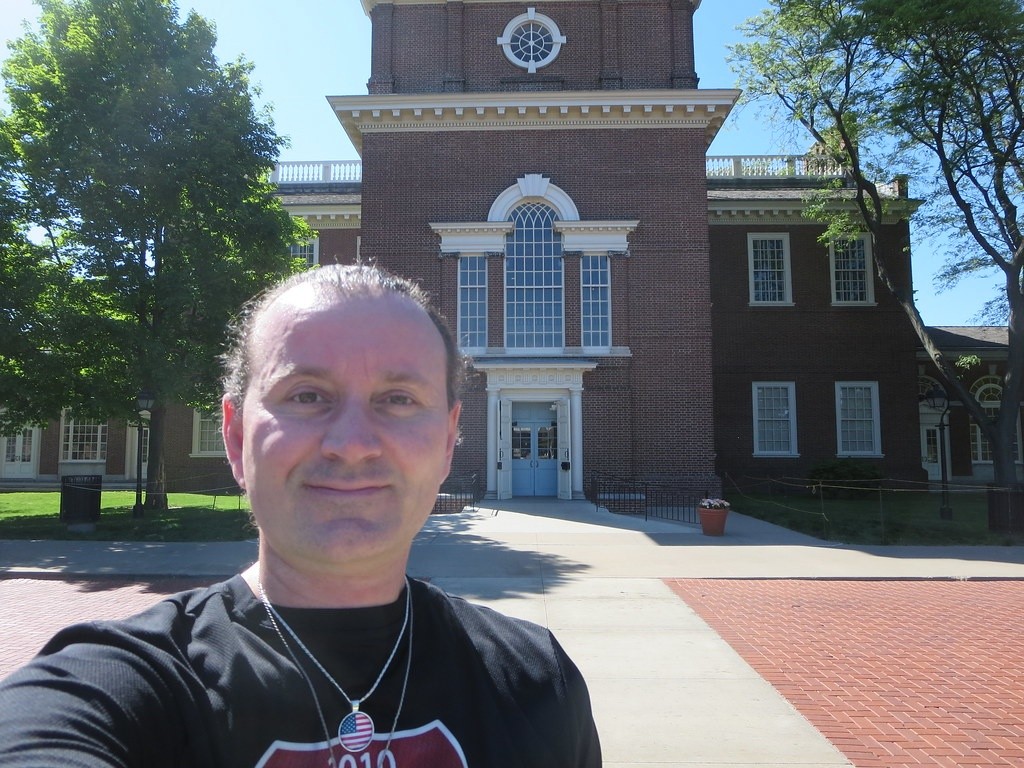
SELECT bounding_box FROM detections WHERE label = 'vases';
[697,508,729,536]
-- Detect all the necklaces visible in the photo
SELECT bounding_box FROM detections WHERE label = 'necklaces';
[257,574,413,768]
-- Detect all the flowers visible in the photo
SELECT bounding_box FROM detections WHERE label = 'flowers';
[698,495,731,510]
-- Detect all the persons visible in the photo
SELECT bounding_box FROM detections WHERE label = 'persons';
[0,264,604,768]
